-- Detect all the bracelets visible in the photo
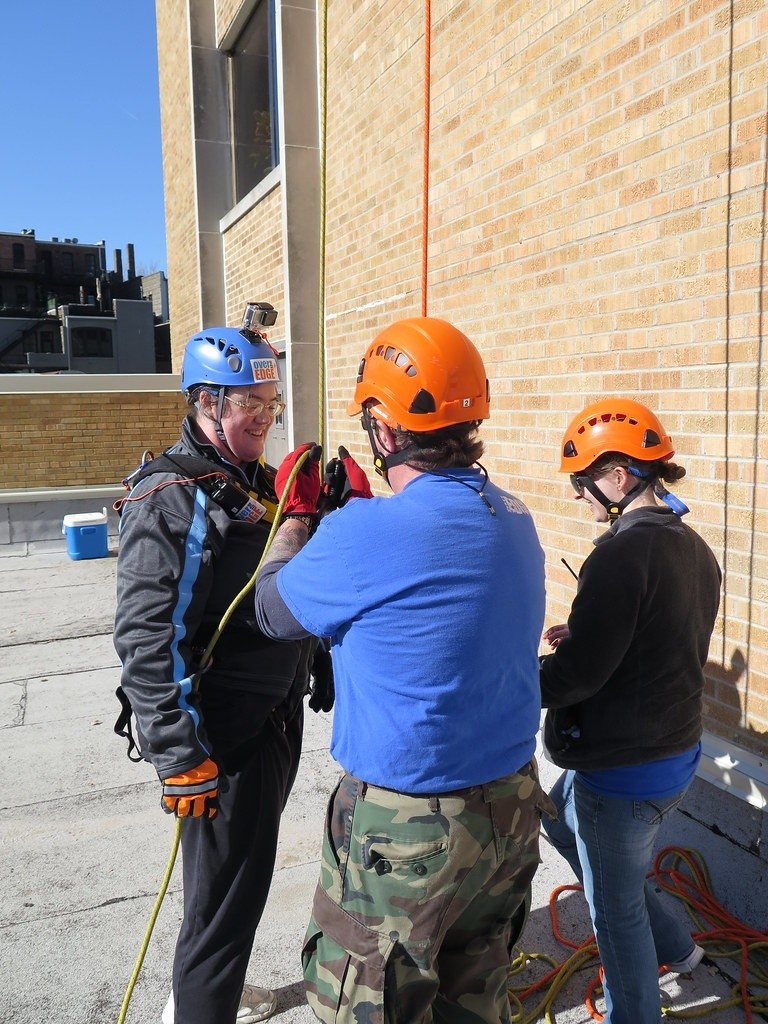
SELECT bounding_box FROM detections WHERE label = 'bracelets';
[281,514,313,534]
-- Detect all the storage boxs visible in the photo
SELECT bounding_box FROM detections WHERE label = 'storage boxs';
[61,507,108,561]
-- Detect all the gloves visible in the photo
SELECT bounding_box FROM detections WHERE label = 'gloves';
[274,442,322,515]
[160,760,218,822]
[322,446,371,510]
[307,653,335,713]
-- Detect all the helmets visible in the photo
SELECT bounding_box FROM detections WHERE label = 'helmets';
[347,317,491,432]
[559,398,676,474]
[181,327,279,395]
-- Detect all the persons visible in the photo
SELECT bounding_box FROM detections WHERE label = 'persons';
[540,397,722,1024]
[255,317,546,1024]
[113,327,336,1024]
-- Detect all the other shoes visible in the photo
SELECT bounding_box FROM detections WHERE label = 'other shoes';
[660,945,704,974]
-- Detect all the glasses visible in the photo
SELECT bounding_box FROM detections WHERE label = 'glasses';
[195,387,285,418]
[358,416,377,430]
[570,465,618,498]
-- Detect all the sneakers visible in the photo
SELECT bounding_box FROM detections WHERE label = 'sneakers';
[162,983,276,1024]
